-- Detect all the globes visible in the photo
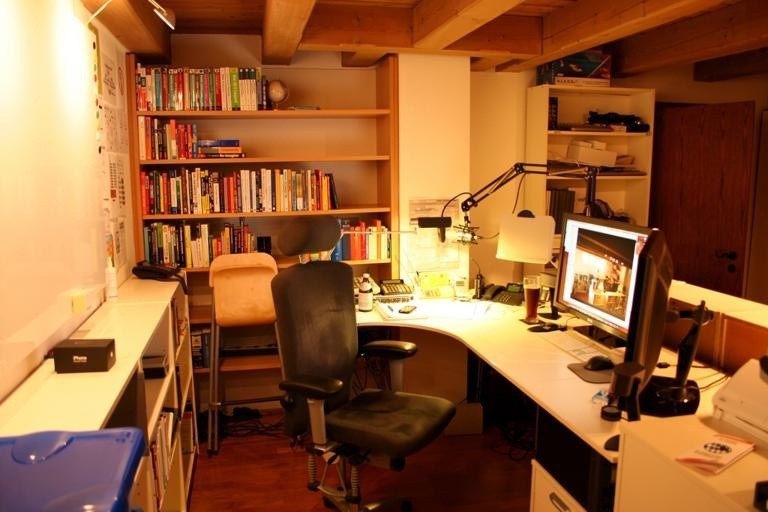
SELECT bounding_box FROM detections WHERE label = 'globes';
[267,80,290,111]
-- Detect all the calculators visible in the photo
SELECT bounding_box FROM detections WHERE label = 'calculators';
[380,279,412,295]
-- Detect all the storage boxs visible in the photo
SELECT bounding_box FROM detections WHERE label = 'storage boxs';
[536,47,612,87]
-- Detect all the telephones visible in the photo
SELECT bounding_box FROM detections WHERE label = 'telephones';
[132,260,175,280]
[484,285,524,306]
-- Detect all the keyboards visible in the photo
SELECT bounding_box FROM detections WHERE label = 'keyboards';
[542,329,609,364]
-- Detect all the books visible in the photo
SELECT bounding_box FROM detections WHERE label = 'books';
[545,187,575,235]
[674,434,756,476]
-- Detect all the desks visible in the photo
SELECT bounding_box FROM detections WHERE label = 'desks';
[356,292,735,511]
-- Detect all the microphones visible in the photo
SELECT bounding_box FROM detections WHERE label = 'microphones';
[446,230,481,242]
[438,192,472,242]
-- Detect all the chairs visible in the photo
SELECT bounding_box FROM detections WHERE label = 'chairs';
[208,253,305,455]
[270,260,456,512]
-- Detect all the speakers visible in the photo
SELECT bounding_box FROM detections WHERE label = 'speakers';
[538,267,561,320]
[605,360,646,452]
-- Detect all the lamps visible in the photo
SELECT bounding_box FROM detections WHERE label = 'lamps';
[417,157,631,319]
[86,0,177,32]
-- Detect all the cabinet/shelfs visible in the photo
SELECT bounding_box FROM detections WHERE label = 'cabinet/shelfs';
[522,84,657,285]
[124,51,401,417]
[0,268,199,512]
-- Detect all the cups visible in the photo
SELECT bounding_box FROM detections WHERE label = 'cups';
[257,235,272,254]
[521,274,542,325]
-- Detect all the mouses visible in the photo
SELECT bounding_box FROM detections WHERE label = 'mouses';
[584,356,615,371]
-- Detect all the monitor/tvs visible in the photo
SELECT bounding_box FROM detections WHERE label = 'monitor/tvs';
[552,212,652,351]
[615,228,714,418]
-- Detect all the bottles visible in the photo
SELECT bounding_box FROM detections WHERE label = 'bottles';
[106,256,118,298]
[474,268,485,300]
[356,273,375,312]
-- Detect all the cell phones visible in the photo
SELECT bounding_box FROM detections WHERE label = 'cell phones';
[399,306,416,313]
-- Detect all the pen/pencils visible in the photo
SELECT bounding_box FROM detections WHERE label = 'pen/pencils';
[388,305,394,312]
